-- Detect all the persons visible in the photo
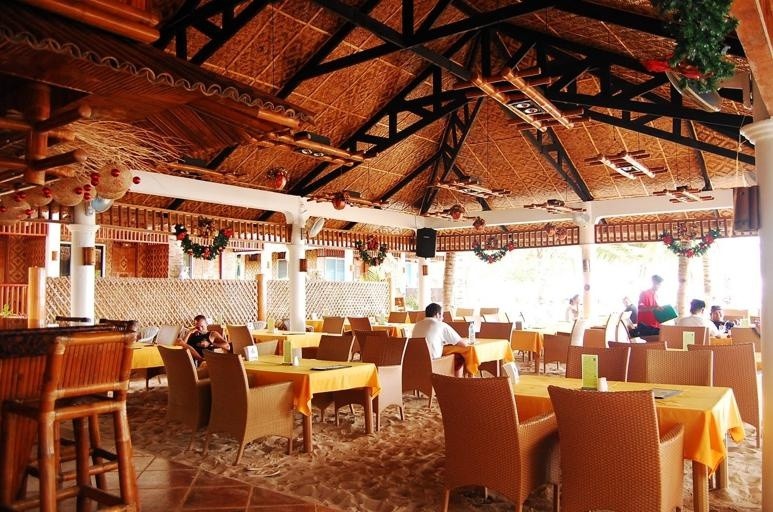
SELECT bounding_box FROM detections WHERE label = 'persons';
[623,296,638,325]
[678,299,727,339]
[175,315,231,370]
[411,302,469,378]
[636,275,664,335]
[565,294,580,322]
[709,305,734,333]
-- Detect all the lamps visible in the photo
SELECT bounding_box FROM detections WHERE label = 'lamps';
[91,195,114,215]
[304,215,326,239]
[268,0,364,168]
[652,141,714,205]
[307,127,389,210]
[426,97,512,199]
[452,0,584,133]
[423,168,476,222]
[523,176,586,215]
[583,29,668,181]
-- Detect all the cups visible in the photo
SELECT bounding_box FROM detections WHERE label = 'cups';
[717,325,726,335]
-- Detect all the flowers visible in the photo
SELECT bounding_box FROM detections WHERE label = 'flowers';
[660,221,722,258]
[471,235,515,263]
[174,216,234,260]
[354,235,389,267]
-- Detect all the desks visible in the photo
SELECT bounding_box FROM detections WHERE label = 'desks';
[242,352,381,453]
[0,313,116,501]
[510,373,747,511]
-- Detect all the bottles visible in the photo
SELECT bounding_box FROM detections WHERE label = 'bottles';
[467,320,475,345]
[27,320,44,328]
[291,355,300,367]
[598,376,607,392]
[28,267,44,320]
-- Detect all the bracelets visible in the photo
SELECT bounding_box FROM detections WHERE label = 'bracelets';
[208,343,213,349]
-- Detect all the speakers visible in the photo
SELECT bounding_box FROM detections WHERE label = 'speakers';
[416,228,436,257]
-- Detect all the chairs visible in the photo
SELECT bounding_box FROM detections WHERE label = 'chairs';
[54,316,92,327]
[201,349,295,467]
[430,372,562,512]
[98,318,138,334]
[444,306,763,449]
[129,311,454,431]
[157,343,210,451]
[0,333,139,511]
[547,383,685,512]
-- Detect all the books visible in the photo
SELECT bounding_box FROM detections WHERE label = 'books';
[647,387,683,400]
[652,304,677,323]
[310,363,354,371]
